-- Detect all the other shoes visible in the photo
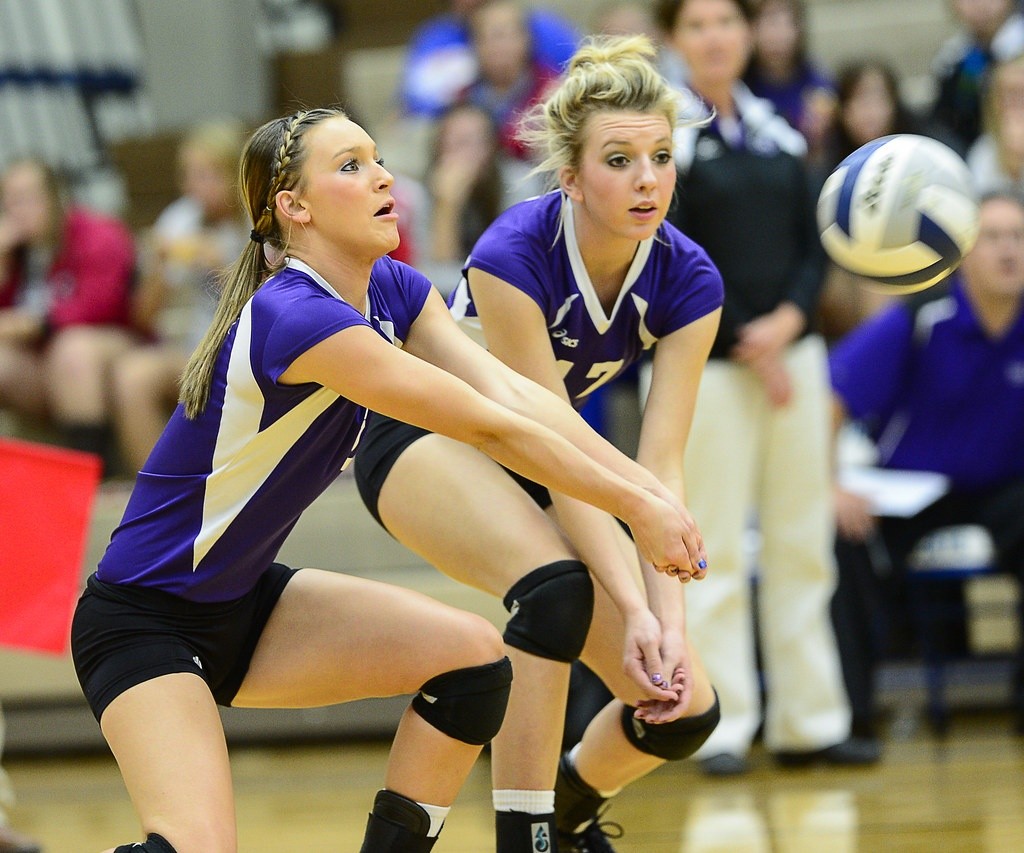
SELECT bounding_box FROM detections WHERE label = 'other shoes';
[776,732,881,769]
[704,753,745,777]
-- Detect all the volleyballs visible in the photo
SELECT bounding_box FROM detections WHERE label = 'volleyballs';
[813,132,984,298]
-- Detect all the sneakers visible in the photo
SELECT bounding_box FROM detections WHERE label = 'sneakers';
[550,802,623,853]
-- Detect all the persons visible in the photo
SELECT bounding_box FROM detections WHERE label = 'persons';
[0,0,1024,853]
